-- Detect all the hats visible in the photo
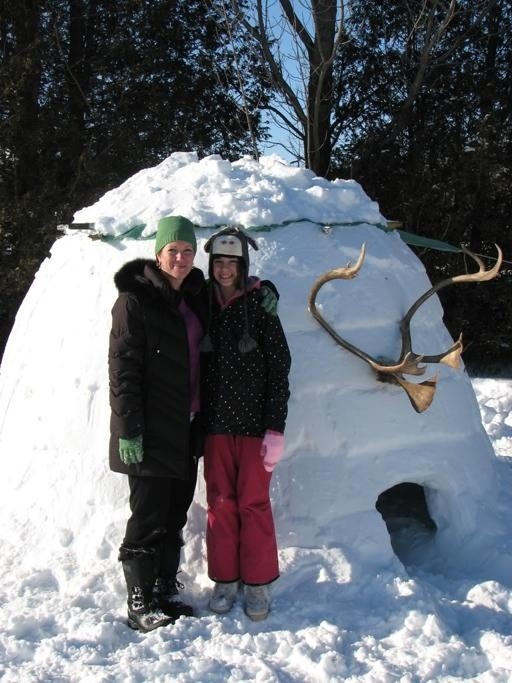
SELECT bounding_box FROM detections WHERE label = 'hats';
[198,226,258,352]
[155,216,197,254]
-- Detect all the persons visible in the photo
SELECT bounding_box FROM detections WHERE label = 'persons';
[108,215,207,634]
[201,225,292,622]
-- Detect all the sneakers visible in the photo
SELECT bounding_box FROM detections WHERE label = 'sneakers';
[244,584,268,621]
[210,580,241,613]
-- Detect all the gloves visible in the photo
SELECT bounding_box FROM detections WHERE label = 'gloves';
[119,434,144,464]
[260,285,278,317]
[260,430,284,472]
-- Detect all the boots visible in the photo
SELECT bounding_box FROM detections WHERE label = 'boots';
[119,546,193,631]
